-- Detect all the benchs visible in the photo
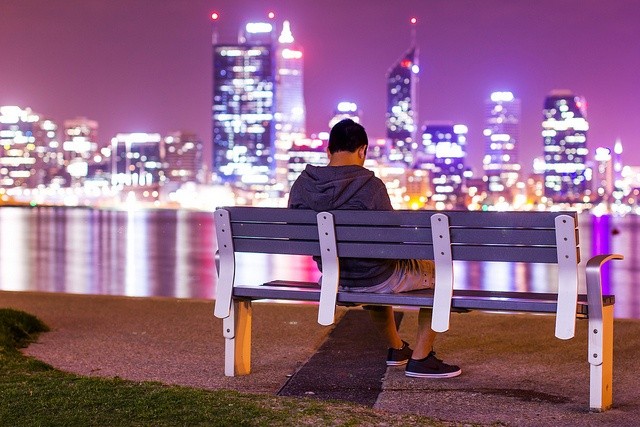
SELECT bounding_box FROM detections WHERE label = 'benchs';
[214,205,624,413]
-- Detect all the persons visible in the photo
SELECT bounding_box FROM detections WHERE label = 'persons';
[288,118,462,378]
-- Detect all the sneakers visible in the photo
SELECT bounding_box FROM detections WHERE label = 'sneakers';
[385,340,414,365]
[404,351,462,379]
